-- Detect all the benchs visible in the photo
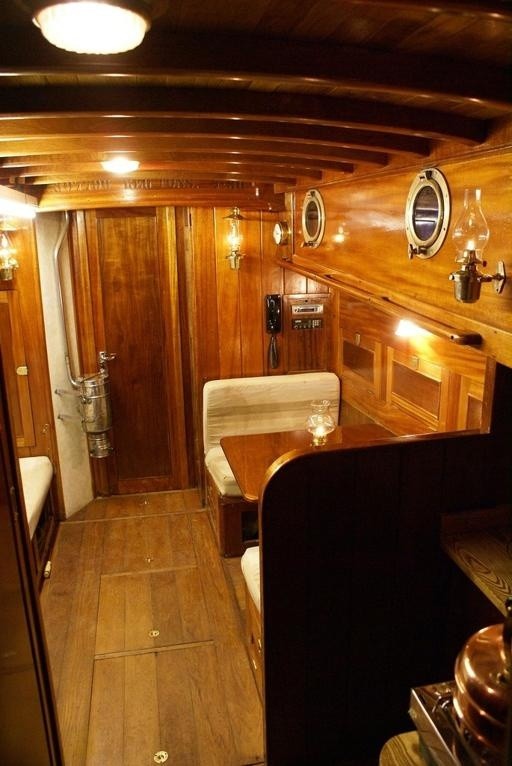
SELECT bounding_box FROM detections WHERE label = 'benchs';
[198,370,484,705]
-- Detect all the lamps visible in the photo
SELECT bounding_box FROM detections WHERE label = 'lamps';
[443,186,507,304]
[222,205,250,273]
[16,1,168,56]
[0,234,21,283]
[102,157,140,173]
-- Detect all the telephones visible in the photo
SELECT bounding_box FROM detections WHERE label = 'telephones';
[266,294,281,334]
